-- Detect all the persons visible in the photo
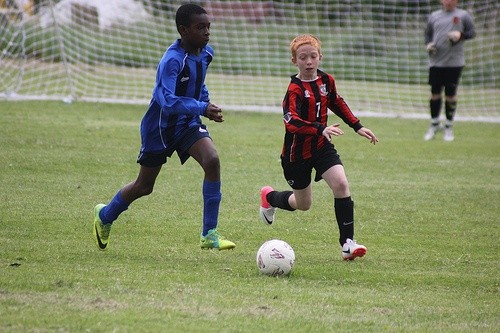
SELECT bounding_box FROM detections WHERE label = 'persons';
[260,35,380,261]
[423,0,475,142]
[94,4,236,252]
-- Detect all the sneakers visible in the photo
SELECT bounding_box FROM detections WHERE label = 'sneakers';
[342,238,367,260]
[201,228,236,250]
[260,186,278,225]
[93,204,112,251]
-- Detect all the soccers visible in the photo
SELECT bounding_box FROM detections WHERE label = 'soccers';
[256,239,296,277]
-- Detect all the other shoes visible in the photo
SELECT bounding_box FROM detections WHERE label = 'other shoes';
[443,121,455,142]
[424,123,443,141]
